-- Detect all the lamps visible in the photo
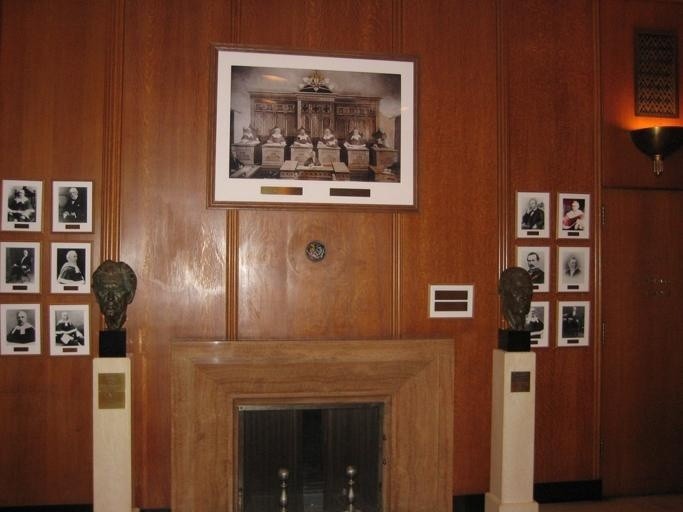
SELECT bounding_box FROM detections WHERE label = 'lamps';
[630,126,682,176]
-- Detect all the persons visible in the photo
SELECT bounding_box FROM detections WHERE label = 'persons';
[89,259,139,329]
[7,185,85,343]
[497,268,536,328]
[239,124,394,147]
[522,197,586,339]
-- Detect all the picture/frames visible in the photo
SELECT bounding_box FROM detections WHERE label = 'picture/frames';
[204,40,422,214]
[0,177,95,358]
[511,189,593,350]
